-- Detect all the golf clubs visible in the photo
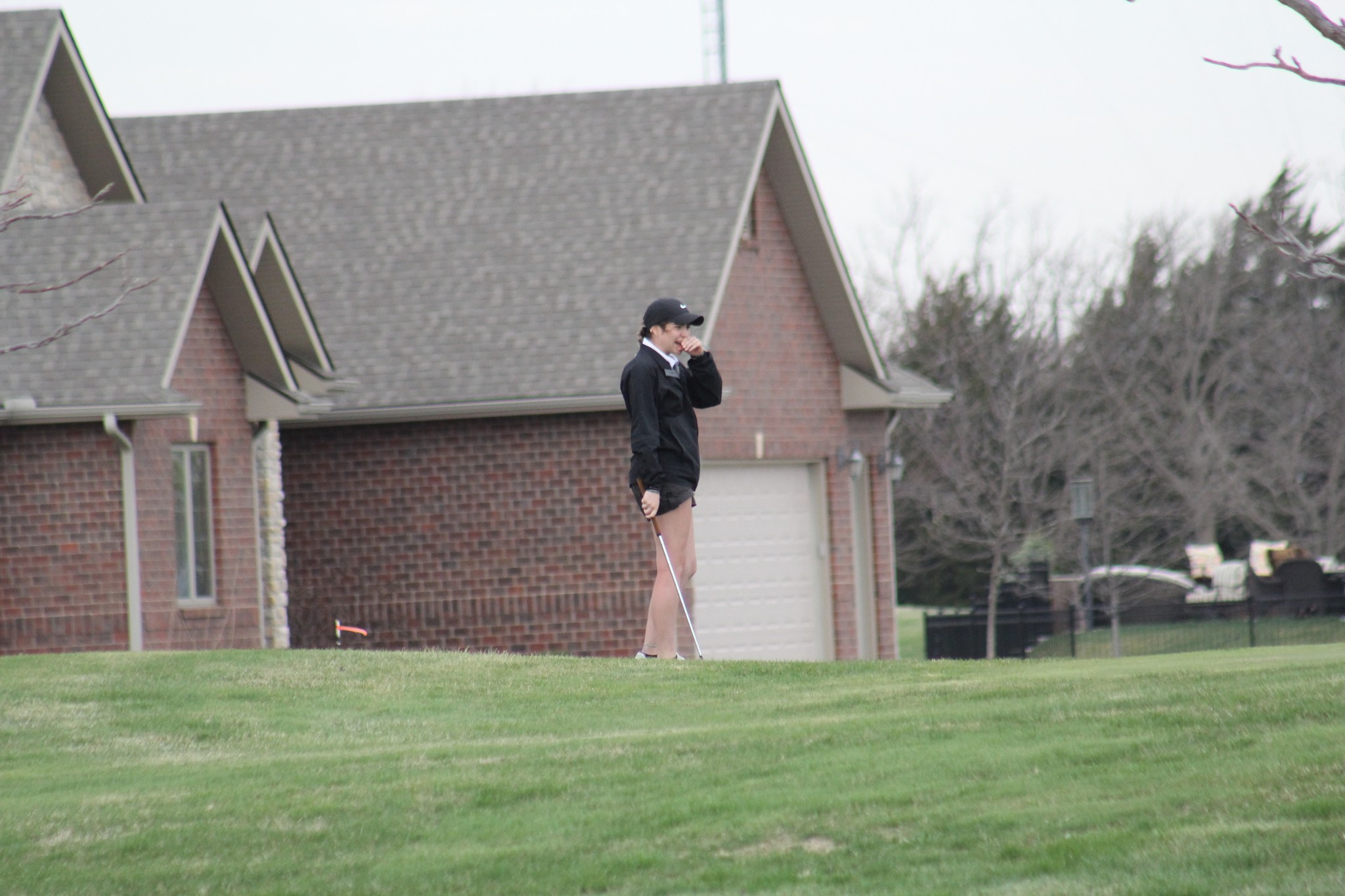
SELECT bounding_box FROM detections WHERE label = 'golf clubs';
[635,477,705,660]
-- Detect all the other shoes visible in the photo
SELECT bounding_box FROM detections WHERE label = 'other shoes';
[676,653,685,660]
[634,651,657,659]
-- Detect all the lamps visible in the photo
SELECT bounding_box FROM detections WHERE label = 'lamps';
[877,447,906,486]
[837,435,865,478]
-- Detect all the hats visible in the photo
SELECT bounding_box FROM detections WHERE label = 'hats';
[642,298,704,328]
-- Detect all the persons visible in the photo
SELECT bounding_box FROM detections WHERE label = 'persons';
[620,297,722,663]
